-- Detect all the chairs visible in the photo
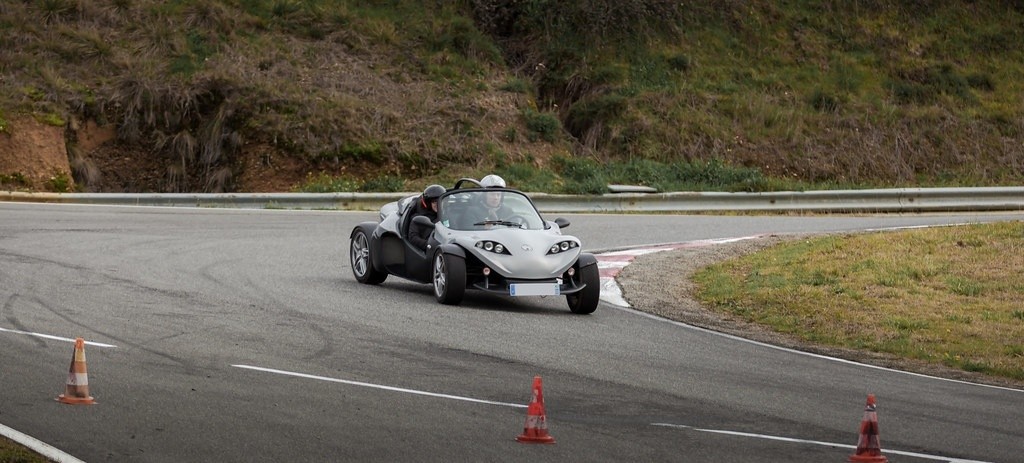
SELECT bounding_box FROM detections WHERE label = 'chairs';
[412,195,426,217]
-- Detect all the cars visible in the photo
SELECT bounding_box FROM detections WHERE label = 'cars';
[348,173,601,317]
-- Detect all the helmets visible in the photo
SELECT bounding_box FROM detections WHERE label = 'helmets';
[481,174,506,188]
[424,185,446,197]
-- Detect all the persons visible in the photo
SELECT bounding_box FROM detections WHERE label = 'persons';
[407,184,457,253]
[461,175,519,229]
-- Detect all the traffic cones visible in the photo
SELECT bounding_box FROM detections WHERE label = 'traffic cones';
[851,392,888,461]
[53,337,98,406]
[514,376,556,445]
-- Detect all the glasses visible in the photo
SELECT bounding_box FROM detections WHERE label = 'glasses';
[432,201,438,204]
